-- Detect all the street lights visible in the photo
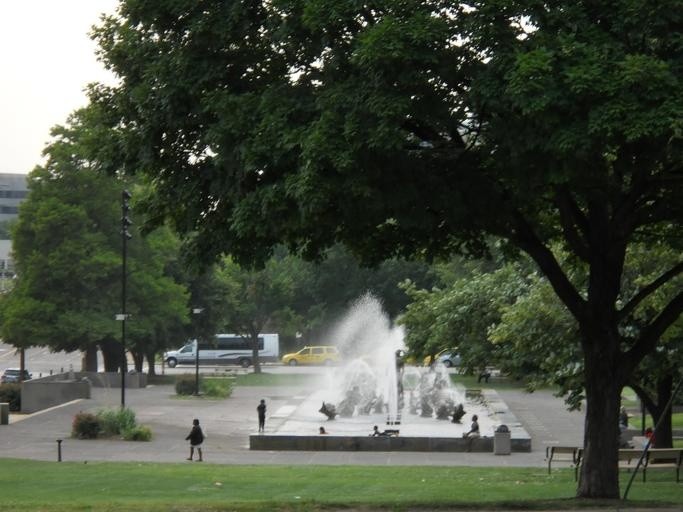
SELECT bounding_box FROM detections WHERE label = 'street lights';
[119,190,132,411]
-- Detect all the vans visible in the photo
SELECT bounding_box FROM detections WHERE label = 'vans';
[283,345,341,365]
[163,334,279,367]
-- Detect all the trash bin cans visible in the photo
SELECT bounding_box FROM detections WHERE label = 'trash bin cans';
[493,422,513,455]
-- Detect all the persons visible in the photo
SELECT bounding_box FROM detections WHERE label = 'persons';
[185,419,204,462]
[464,414,480,451]
[373,426,381,436]
[620,405,628,430]
[318,427,328,434]
[257,400,266,432]
[644,427,656,448]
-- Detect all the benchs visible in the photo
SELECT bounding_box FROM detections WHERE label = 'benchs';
[544,446,683,482]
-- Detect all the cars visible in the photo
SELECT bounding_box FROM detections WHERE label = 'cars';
[1,369,33,384]
[423,347,464,367]
